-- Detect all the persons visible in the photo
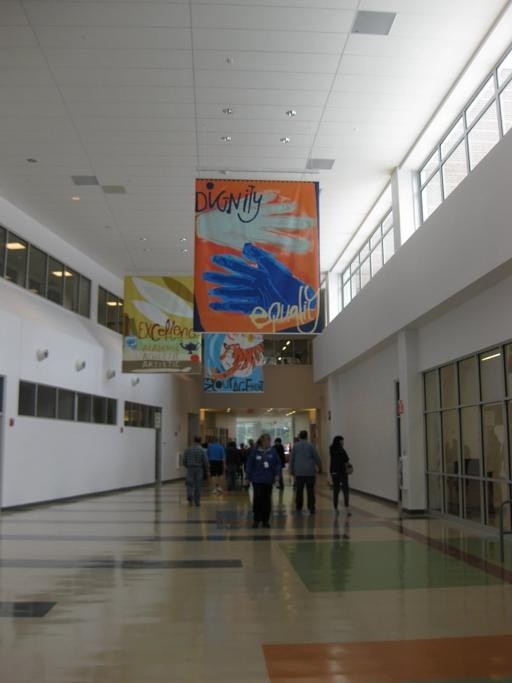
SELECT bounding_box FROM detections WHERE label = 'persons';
[244,433,282,528]
[330,512,356,594]
[328,435,354,517]
[432,428,512,524]
[287,431,323,515]
[245,525,285,612]
[179,435,299,506]
[291,510,326,591]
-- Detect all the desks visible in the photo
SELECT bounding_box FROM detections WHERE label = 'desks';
[448,471,496,514]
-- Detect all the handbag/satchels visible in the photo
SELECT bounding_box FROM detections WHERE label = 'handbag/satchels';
[345,463,353,474]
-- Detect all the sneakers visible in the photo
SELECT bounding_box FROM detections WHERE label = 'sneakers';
[334,506,351,516]
[296,505,315,514]
[188,498,200,506]
[253,521,271,529]
[212,486,223,494]
